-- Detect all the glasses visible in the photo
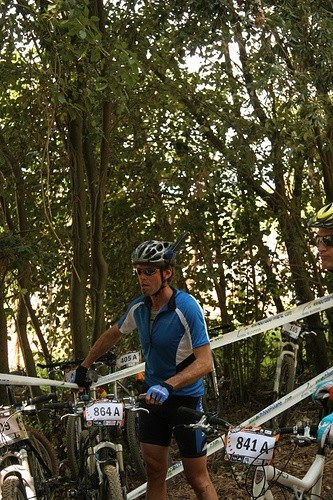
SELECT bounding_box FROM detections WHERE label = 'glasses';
[315,235,333,247]
[134,267,159,276]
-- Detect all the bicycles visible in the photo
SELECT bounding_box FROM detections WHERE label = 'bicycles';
[0,346,333,500]
[200,322,234,417]
[268,312,333,429]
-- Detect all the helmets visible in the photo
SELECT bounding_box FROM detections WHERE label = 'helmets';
[132,241,174,266]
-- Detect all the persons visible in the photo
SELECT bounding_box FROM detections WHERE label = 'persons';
[72,239,220,500]
[314,203,333,450]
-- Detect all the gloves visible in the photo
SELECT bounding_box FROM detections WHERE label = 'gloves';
[312,378,333,405]
[316,412,333,448]
